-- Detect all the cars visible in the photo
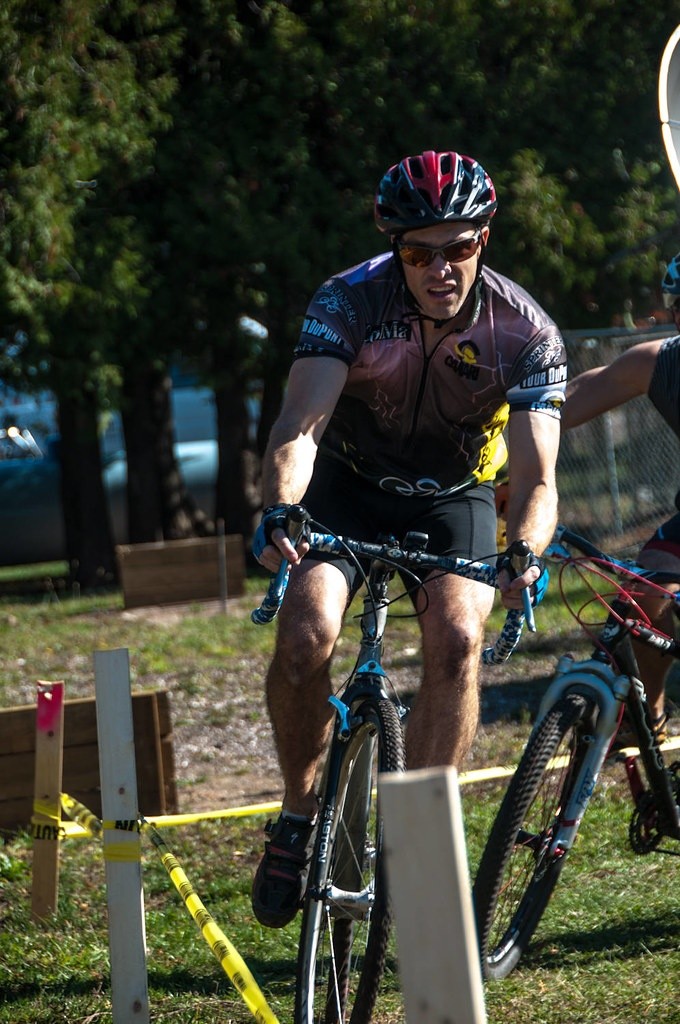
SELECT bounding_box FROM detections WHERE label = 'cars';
[0,315,290,566]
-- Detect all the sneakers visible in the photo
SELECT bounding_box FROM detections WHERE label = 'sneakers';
[252,811,321,928]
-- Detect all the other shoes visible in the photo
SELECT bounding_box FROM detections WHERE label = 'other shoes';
[613,702,670,748]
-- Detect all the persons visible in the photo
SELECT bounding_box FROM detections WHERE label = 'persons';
[496,251,680,763]
[248,152,566,927]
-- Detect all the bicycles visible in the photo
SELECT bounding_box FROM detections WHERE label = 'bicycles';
[245,502,539,1024]
[470,505,680,983]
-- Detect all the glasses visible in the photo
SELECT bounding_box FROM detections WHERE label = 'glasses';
[396,229,481,268]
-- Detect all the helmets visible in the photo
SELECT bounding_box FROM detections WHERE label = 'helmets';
[373,149,498,237]
[661,251,680,294]
